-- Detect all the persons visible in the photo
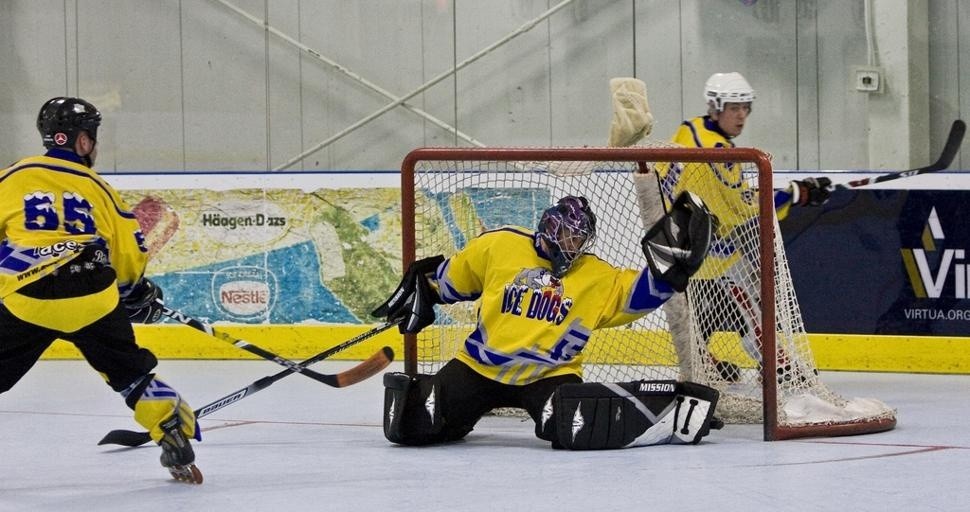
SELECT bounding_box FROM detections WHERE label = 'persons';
[650,71,852,405]
[370,196,719,449]
[0,96,202,468]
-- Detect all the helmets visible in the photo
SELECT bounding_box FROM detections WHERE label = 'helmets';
[703,72,756,112]
[37,97,101,148]
[535,196,597,278]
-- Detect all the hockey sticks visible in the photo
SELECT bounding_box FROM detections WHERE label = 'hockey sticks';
[97,314,404,445]
[155,299,394,387]
[827,120,966,194]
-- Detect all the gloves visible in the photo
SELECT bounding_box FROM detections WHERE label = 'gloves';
[118,278,163,323]
[790,177,832,207]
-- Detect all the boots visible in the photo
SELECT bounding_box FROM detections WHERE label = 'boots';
[160,412,194,466]
